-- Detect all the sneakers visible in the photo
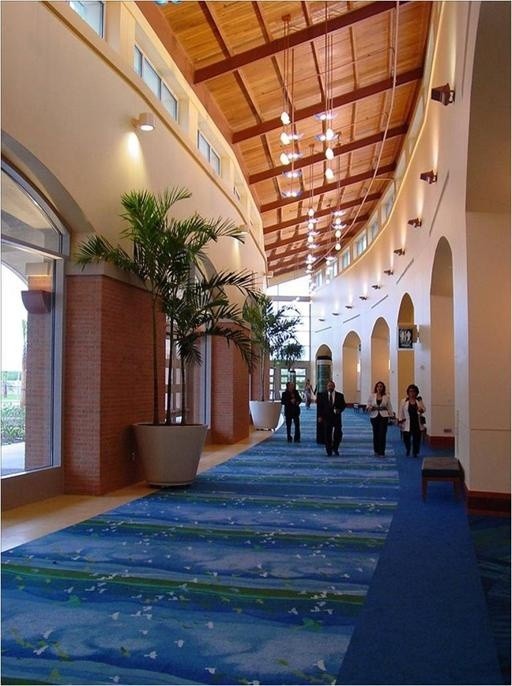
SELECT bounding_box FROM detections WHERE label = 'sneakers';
[327,453,332,456]
[374,452,379,456]
[287,438,292,443]
[294,439,300,442]
[413,452,418,457]
[381,453,385,457]
[334,451,339,455]
[406,452,411,456]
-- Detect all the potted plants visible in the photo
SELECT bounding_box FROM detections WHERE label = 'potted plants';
[72,184,270,486]
[241,297,303,431]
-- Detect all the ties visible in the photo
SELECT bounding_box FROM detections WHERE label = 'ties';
[330,392,333,406]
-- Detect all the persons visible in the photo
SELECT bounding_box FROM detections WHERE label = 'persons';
[313,384,317,396]
[365,381,395,457]
[281,380,302,444]
[397,383,426,459]
[303,378,314,410]
[317,381,346,458]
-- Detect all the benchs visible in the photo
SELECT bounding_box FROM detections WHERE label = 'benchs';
[389,412,396,425]
[422,456,460,504]
[353,403,366,413]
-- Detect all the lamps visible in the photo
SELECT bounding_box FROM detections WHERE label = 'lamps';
[431,85,454,105]
[239,225,248,234]
[133,112,156,132]
[264,270,273,277]
[394,248,405,256]
[18,290,53,315]
[420,171,437,184]
[318,268,394,323]
[408,218,421,228]
[281,0,345,276]
